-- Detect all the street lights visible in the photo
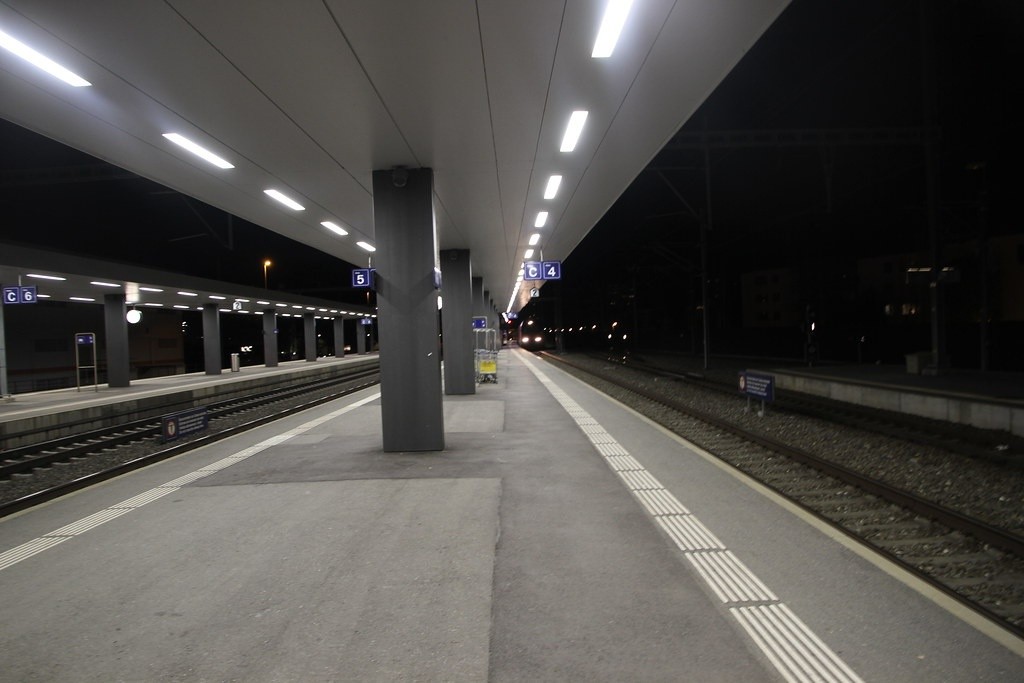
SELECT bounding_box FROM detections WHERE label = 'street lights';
[697,305,709,369]
[906,265,955,369]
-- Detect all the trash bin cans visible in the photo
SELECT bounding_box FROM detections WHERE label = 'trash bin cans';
[231,353,240,372]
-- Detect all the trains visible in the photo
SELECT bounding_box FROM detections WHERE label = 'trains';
[518,319,546,350]
